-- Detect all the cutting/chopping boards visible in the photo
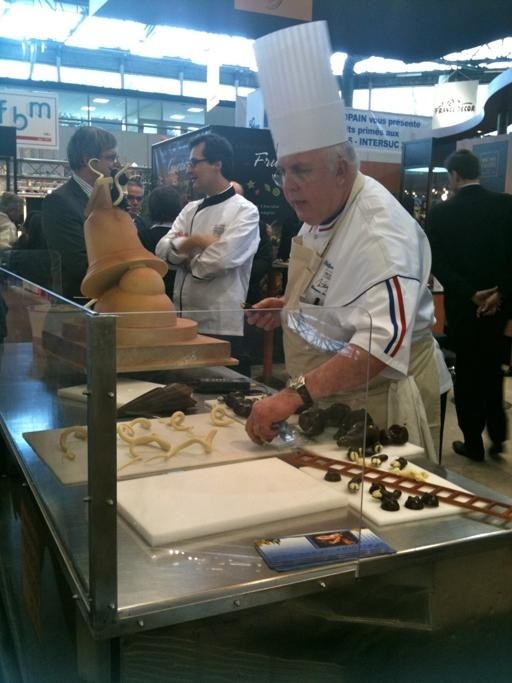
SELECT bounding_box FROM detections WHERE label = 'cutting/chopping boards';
[57,376,166,408]
[299,452,486,529]
[202,393,425,459]
[22,412,292,486]
[116,455,348,547]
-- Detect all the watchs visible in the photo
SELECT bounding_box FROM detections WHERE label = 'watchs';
[286,376,312,408]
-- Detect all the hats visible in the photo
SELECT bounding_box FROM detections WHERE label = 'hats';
[252,21,348,158]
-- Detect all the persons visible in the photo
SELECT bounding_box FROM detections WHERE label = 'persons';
[228,180,271,378]
[40,126,125,301]
[244,20,453,476]
[156,133,261,378]
[0,191,27,255]
[146,184,185,248]
[126,178,156,255]
[419,149,512,460]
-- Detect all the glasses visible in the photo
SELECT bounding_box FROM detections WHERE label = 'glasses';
[183,158,208,167]
[101,154,120,164]
[271,163,333,177]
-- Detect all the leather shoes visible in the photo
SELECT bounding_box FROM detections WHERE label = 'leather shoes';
[453,440,485,460]
[488,442,504,457]
